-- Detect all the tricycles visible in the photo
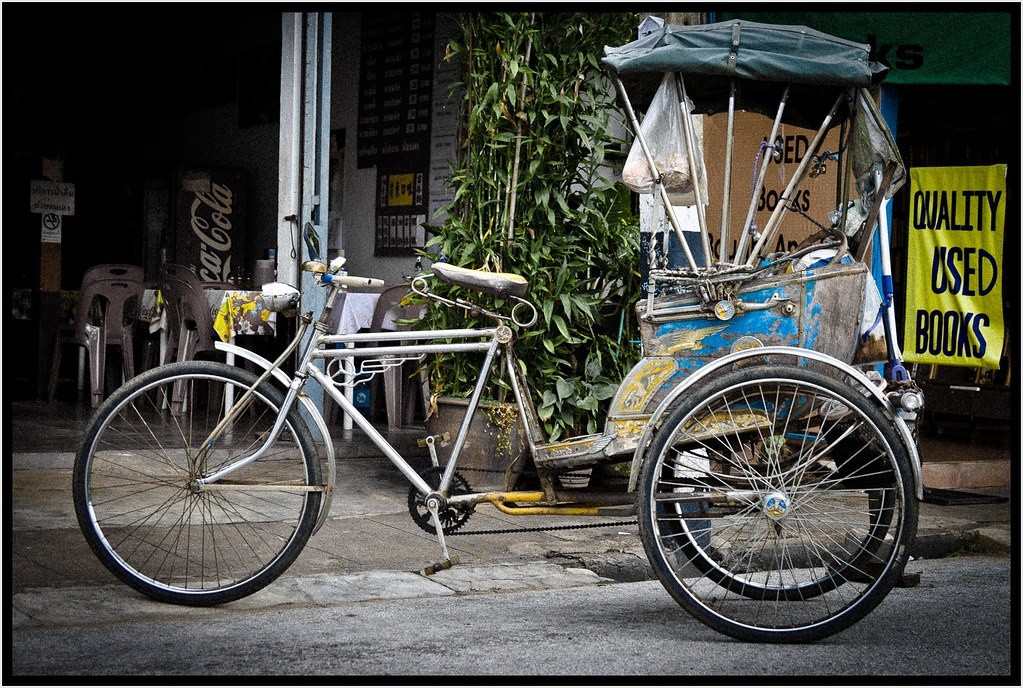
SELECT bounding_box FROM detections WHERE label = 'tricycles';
[71,17,921,641]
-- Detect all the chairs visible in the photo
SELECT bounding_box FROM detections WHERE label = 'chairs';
[323,285,434,432]
[43,261,258,428]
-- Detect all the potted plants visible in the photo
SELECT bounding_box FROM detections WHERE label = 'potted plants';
[394,0,650,492]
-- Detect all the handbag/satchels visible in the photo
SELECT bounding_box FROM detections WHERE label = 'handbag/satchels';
[852,88,908,204]
[622,72,692,193]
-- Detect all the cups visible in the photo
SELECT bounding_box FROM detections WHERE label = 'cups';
[254,260,273,289]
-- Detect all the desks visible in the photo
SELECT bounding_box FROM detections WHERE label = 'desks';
[129,287,277,419]
[325,288,431,441]
[12,288,165,391]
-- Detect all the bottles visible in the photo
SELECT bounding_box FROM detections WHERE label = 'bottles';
[413,255,423,274]
[155,248,168,275]
[337,250,348,290]
